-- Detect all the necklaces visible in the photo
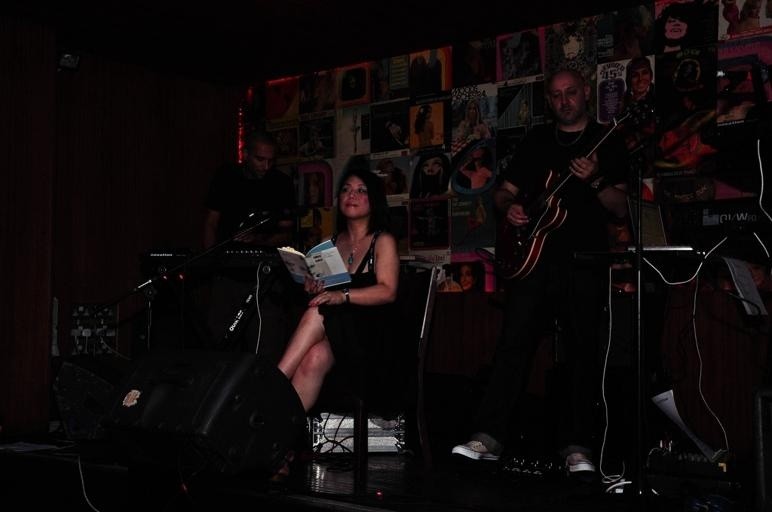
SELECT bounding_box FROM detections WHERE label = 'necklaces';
[346,235,363,265]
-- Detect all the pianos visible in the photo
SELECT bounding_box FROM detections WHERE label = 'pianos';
[139,245,277,283]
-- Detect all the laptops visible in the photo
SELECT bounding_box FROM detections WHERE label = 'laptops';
[625,195,694,252]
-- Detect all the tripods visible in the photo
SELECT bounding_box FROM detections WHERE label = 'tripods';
[553,80,745,498]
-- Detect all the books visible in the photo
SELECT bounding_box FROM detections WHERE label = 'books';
[275,239,353,291]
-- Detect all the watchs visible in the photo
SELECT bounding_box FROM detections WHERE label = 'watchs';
[588,174,605,189]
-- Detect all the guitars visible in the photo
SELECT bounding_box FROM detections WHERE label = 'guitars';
[494,99,645,282]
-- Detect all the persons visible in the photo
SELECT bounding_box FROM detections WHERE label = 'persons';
[448,65,634,471]
[264,3,771,241]
[275,165,400,415]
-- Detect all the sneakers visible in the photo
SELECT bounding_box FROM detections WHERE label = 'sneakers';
[566,452,596,473]
[452,440,500,460]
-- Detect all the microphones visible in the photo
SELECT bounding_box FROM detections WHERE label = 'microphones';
[251,205,309,219]
[716,68,770,81]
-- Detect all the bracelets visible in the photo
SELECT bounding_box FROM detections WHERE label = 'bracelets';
[343,285,350,303]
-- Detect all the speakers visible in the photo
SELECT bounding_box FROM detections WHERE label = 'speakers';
[105,350,314,493]
[51,353,130,441]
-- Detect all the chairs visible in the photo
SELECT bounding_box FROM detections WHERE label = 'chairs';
[306,260,439,499]
